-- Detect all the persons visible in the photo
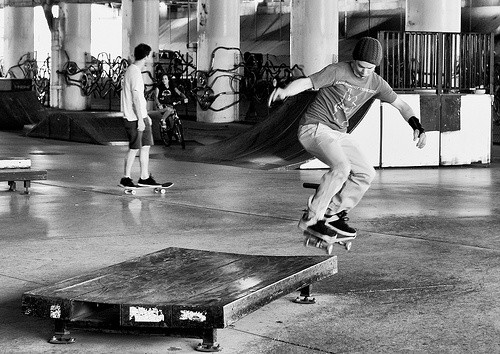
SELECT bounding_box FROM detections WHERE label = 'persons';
[118,43,162,189]
[155,73,188,129]
[268,37,427,239]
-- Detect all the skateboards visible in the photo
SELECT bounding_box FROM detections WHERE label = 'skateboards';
[117,182,174,196]
[304,230,356,256]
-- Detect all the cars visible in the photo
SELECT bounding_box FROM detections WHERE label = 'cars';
[159,49,183,79]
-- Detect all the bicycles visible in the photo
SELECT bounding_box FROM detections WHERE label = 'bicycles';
[160,99,186,149]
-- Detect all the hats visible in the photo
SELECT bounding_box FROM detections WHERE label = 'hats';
[353,37,382,66]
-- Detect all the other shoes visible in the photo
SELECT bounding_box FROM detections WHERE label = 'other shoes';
[160,119,167,128]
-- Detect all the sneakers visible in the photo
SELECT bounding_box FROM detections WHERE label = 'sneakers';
[138,176,162,187]
[120,177,137,189]
[297,213,337,241]
[325,211,356,236]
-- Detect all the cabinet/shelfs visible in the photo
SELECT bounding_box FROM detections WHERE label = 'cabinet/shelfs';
[382,94,441,167]
[440,94,492,165]
[299,98,381,170]
